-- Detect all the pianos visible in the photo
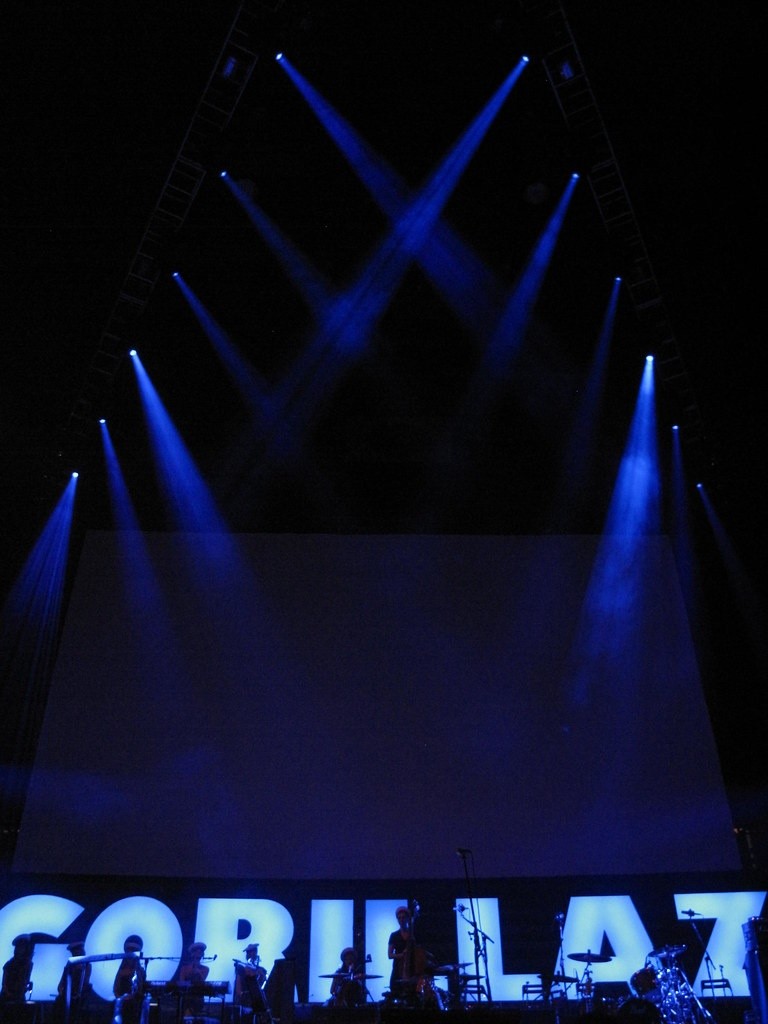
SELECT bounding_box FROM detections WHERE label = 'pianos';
[144,979,233,996]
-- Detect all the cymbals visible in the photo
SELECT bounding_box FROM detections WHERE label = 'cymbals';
[444,973,486,981]
[318,969,350,979]
[567,948,613,963]
[351,973,383,980]
[538,971,580,983]
[458,962,473,968]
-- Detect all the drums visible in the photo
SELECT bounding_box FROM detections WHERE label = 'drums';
[628,966,668,1004]
[611,995,661,1024]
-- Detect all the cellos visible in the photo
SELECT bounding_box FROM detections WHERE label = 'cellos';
[393,899,422,999]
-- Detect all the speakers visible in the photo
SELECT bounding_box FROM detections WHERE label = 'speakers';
[743,918,768,1024]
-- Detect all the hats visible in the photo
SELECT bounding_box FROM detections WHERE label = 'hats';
[396,907,411,920]
[244,944,258,952]
[188,942,205,951]
[12,935,35,947]
[341,949,357,961]
[67,941,83,950]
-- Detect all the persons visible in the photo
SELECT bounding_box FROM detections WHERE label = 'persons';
[0,940,34,1001]
[330,947,358,1007]
[57,945,92,996]
[113,935,146,999]
[180,943,209,1016]
[388,907,418,989]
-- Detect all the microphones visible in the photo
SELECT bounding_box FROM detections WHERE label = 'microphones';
[453,906,469,910]
[213,954,217,960]
[455,848,468,859]
[555,914,564,920]
[367,954,372,962]
[682,911,702,915]
